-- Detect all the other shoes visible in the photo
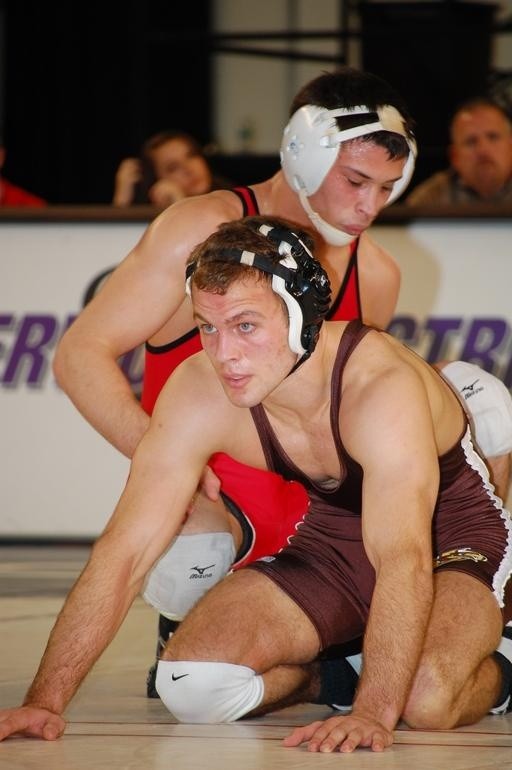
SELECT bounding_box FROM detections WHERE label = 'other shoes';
[322,650,360,711]
[488,621,512,714]
[147,635,170,698]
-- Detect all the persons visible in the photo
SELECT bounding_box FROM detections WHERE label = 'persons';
[48,67,512,707]
[110,131,215,210]
[0,213,512,756]
[401,93,512,209]
